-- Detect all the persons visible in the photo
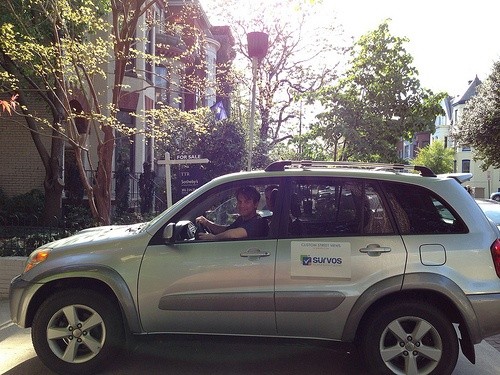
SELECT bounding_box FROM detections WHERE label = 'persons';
[195,186,270,242]
[262,184,280,225]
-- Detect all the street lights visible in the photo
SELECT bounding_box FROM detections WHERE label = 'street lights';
[246,31,269,171]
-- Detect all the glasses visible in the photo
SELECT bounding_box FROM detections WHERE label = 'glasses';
[239,187,259,197]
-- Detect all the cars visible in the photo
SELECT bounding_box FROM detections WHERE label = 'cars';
[490,190,500,201]
[433,199,500,229]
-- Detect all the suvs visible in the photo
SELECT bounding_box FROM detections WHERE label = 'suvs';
[8,158,500,374]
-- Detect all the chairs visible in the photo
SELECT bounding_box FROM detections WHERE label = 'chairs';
[355,194,371,233]
[267,188,279,239]
[290,192,304,233]
